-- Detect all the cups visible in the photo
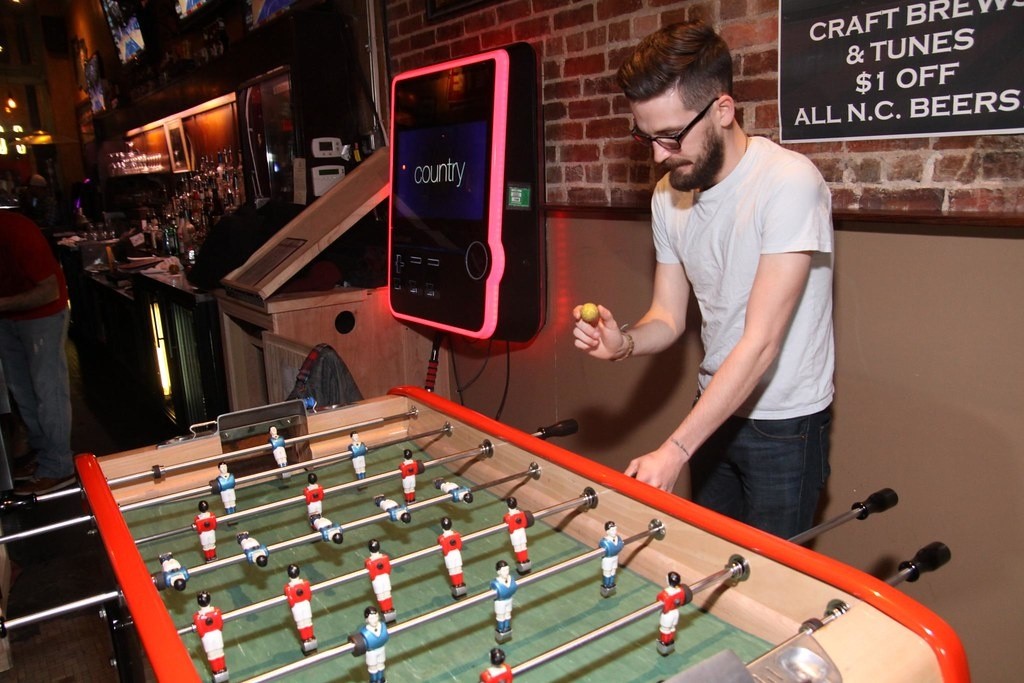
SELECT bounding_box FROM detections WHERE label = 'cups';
[105,150,164,176]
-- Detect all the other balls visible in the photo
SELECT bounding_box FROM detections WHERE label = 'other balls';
[580,303,598,322]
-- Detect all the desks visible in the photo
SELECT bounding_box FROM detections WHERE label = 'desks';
[73,378,972,683]
[86,269,229,435]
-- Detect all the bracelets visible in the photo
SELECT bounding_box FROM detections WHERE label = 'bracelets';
[609,324,635,363]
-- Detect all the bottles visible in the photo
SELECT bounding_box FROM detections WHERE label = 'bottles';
[85,222,116,241]
[198,17,229,66]
[142,144,247,264]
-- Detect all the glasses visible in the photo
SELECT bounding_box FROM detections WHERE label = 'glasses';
[629,97,720,150]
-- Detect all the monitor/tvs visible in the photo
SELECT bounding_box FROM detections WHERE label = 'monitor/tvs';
[98,1,152,70]
[166,0,229,33]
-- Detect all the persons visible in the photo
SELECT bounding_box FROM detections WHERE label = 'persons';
[0,173,78,496]
[569,17,836,548]
[431,66,469,103]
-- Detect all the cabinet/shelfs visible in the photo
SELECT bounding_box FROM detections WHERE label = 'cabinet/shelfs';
[210,293,452,409]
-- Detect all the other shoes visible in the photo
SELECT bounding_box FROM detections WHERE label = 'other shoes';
[12,463,37,480]
[13,472,77,496]
